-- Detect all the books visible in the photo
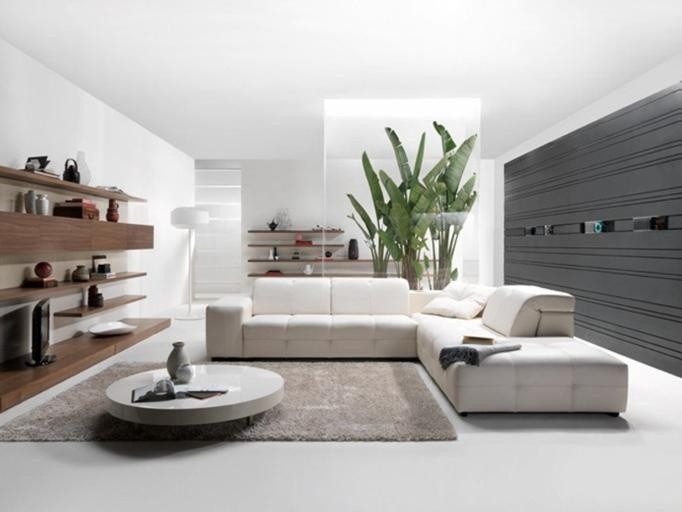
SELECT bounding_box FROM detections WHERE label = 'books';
[463,335,495,345]
[131,378,228,402]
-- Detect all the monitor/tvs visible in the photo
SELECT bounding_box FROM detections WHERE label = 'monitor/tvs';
[24,297,52,367]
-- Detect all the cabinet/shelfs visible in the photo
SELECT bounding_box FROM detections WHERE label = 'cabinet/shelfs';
[247,229,443,277]
[1,163,172,424]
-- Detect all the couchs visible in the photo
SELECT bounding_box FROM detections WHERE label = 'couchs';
[206,274,628,416]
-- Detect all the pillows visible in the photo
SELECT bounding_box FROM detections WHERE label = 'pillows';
[421,279,496,321]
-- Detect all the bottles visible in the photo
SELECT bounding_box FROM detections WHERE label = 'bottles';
[88,284,103,307]
[166,340,194,383]
[72,265,90,282]
[24,191,49,216]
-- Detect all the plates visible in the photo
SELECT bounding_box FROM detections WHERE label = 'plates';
[90,321,137,335]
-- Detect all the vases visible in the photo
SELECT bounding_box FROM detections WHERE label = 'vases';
[166,342,189,380]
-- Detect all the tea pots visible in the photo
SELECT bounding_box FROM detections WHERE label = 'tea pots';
[301,263,315,275]
[267,220,279,231]
[64,158,80,182]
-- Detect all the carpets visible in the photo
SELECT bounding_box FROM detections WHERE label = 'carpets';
[1,360,459,443]
